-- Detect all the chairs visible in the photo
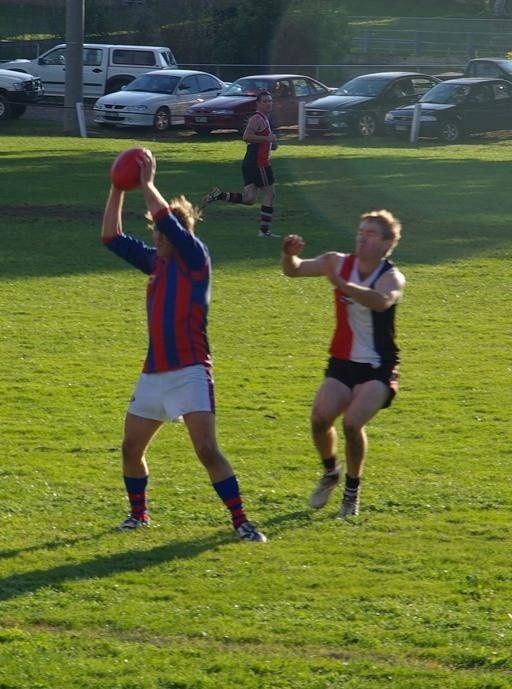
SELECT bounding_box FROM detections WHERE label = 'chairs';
[258,229,282,238]
[338,490,362,518]
[199,188,221,207]
[122,517,149,531]
[235,522,265,542]
[310,468,340,508]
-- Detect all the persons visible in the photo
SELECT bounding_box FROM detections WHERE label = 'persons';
[203,89,282,238]
[103,152,266,545]
[283,210,406,519]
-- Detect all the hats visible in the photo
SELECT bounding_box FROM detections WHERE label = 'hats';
[184,75,338,137]
[304,72,443,137]
[92,71,242,134]
[384,78,511,142]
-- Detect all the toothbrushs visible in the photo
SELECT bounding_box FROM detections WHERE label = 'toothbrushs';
[110,146,154,192]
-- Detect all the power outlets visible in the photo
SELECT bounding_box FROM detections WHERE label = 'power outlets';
[432,59,512,80]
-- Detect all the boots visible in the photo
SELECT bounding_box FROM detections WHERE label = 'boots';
[0,68,44,121]
[0,45,178,99]
